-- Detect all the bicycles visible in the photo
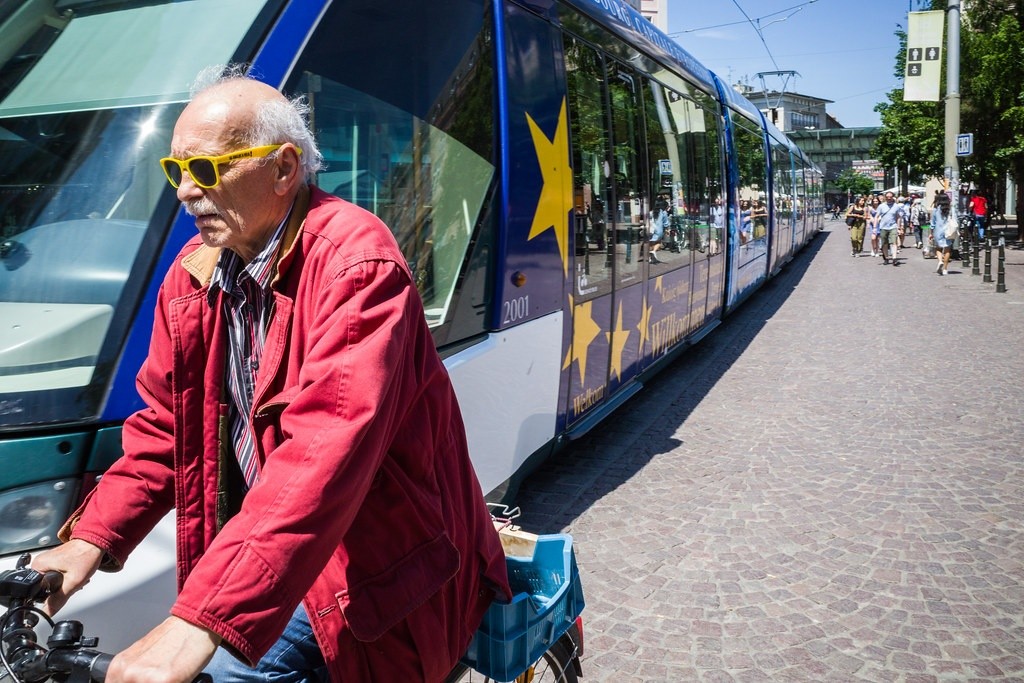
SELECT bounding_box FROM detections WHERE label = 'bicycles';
[1,515,593,683]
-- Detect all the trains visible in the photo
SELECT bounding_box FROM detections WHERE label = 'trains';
[0,0,822,683]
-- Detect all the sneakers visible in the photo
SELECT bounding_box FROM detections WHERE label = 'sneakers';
[936,261,948,276]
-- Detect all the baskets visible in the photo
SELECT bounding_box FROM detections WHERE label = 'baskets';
[453,509,585,683]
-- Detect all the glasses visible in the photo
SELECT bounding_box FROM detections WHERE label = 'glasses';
[159,144,304,193]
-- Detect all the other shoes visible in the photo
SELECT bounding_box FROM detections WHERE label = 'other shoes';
[851,240,987,266]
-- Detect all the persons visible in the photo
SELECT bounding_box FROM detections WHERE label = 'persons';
[27,66,515,683]
[577,182,824,257]
[831,189,989,277]
